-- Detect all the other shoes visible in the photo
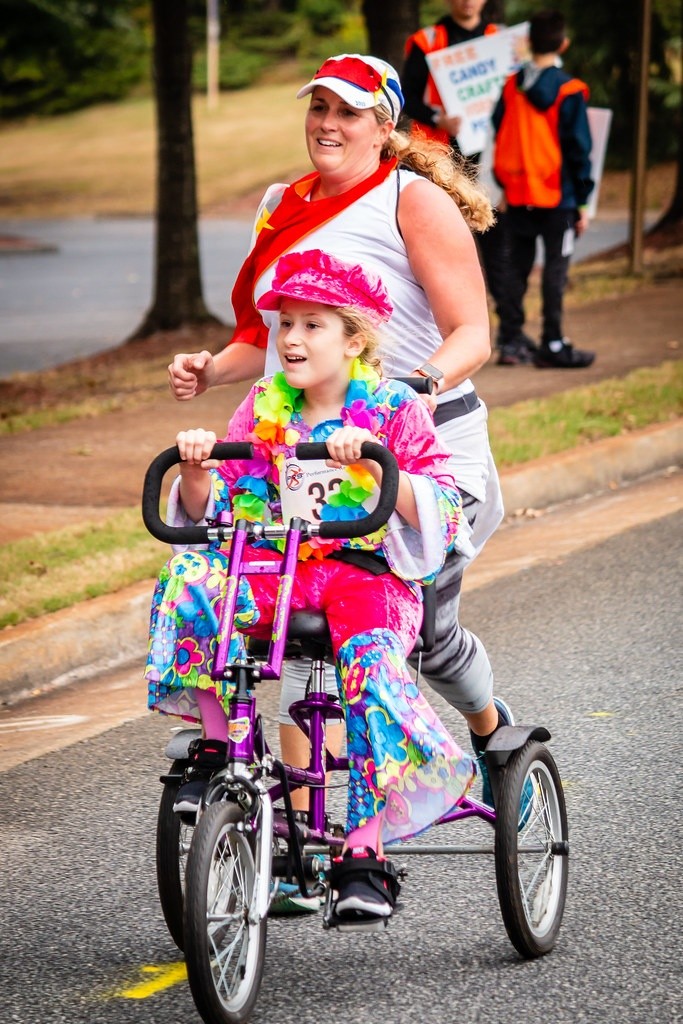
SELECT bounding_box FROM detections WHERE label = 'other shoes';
[495,338,538,364]
[532,338,597,369]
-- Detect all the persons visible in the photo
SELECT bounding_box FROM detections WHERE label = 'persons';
[146,249,466,922]
[483,9,598,369]
[168,51,537,911]
[400,0,537,347]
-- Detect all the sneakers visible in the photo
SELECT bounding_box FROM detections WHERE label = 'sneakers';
[267,853,339,918]
[173,731,231,815]
[332,845,402,922]
[468,698,537,834]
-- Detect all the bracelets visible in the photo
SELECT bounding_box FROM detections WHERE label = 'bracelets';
[575,202,589,209]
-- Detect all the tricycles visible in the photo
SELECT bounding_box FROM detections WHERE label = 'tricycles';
[140,441,575,1023]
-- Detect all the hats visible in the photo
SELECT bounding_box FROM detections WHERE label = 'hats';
[296,53,404,130]
[255,249,393,329]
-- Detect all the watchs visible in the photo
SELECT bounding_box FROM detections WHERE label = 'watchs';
[412,364,449,393]
